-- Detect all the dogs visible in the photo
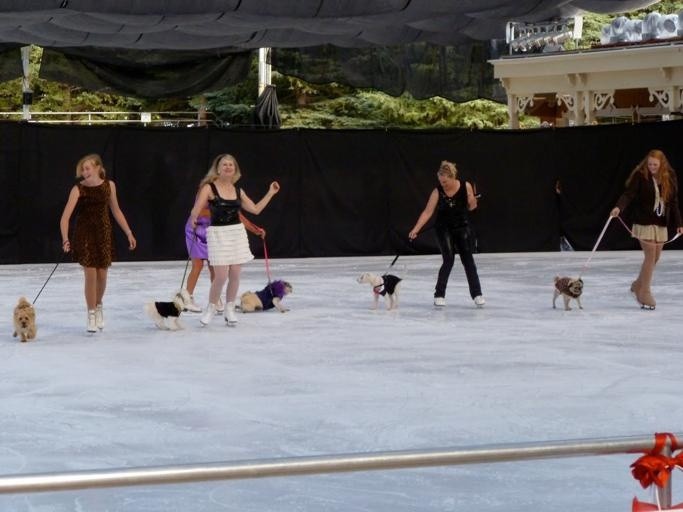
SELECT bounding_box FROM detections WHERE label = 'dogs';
[13,296,37,343]
[552,276,584,311]
[144,288,191,331]
[356,272,403,311]
[235,280,293,313]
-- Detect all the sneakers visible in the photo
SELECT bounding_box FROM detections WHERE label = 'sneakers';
[632,281,656,306]
[434,297,445,306]
[474,295,485,304]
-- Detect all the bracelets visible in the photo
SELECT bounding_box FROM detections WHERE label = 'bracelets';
[125,230,132,236]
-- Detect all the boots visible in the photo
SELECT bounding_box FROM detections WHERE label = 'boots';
[88,304,103,332]
[187,296,237,324]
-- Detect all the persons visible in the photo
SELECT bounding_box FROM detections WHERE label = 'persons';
[407,162,487,306]
[608,149,683,308]
[189,154,281,324]
[58,154,137,333]
[183,173,265,313]
